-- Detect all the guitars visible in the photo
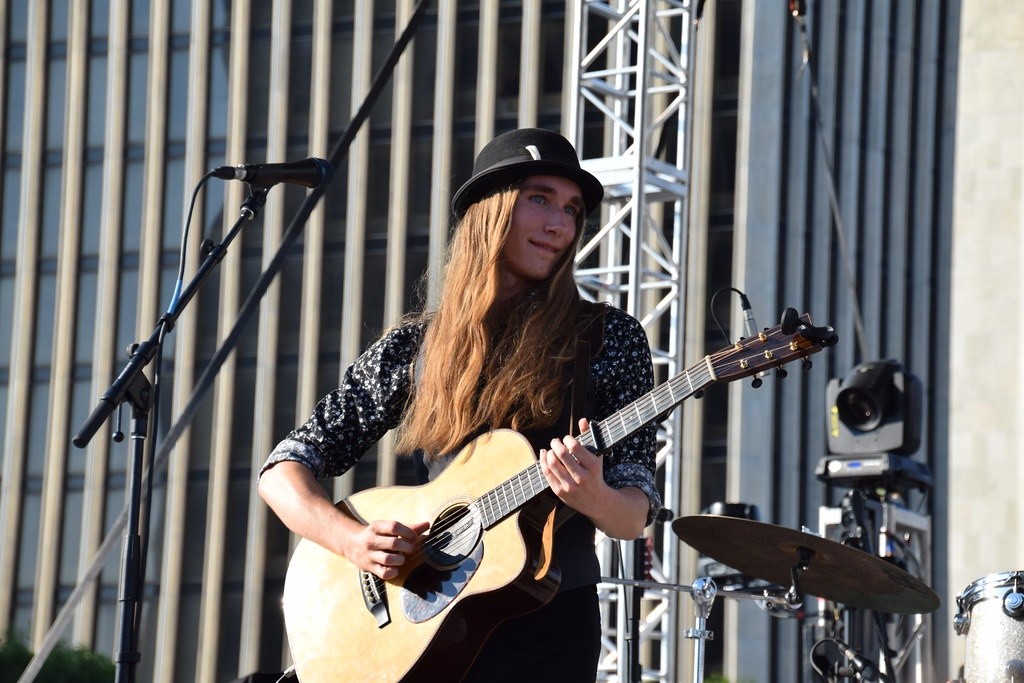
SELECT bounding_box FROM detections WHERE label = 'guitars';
[281,308,838,683]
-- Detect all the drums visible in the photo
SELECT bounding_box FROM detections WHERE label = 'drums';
[951,570,1024,683]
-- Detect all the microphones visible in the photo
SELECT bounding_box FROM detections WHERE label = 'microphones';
[740,294,771,377]
[208,156,335,189]
[834,639,879,682]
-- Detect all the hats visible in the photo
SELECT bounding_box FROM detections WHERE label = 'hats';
[450,125,605,224]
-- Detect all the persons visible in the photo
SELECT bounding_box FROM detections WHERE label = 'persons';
[255,127,657,682]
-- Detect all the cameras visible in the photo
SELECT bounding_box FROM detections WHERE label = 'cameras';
[824,356,922,459]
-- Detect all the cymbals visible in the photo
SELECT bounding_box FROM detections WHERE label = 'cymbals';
[671,515,942,616]
